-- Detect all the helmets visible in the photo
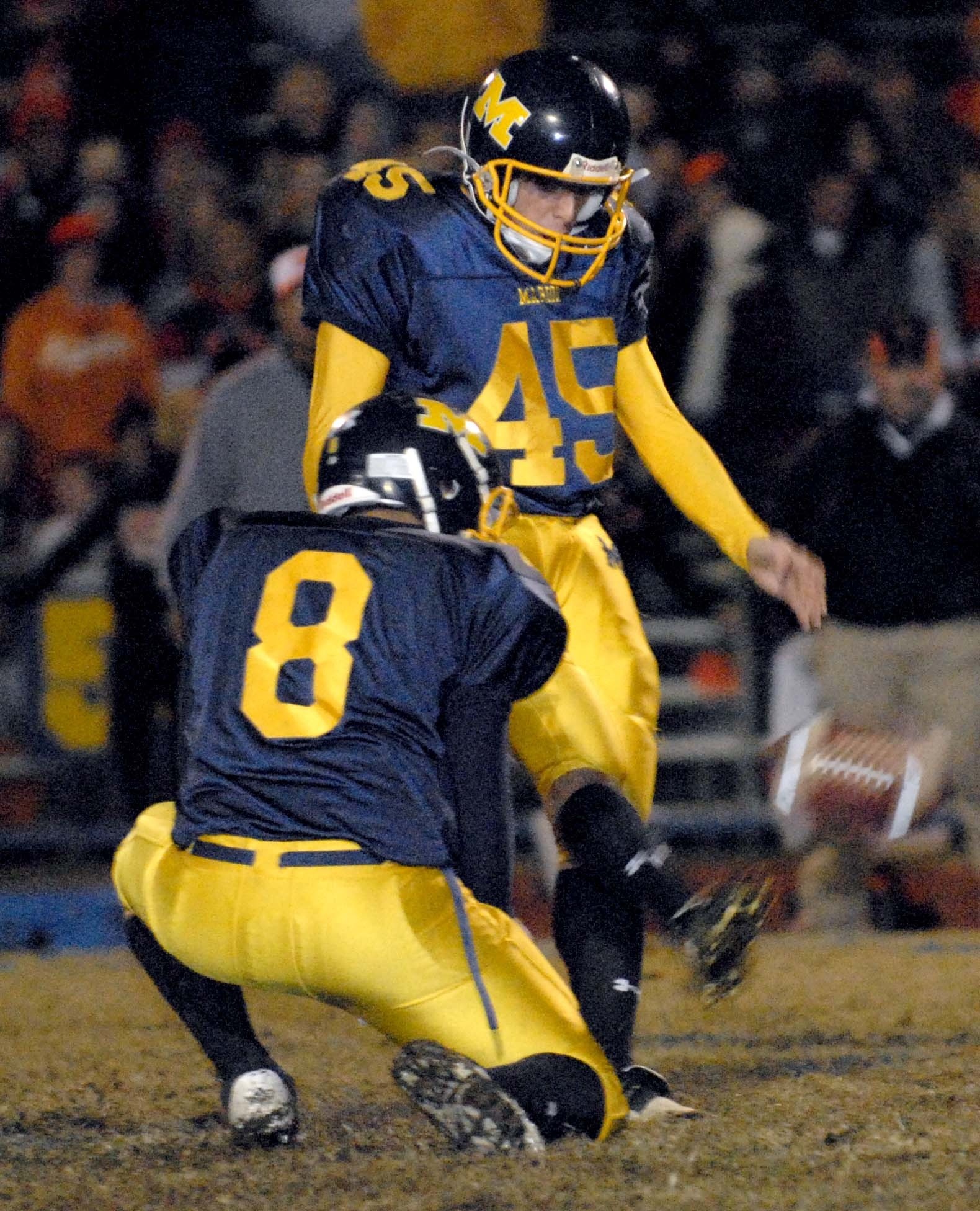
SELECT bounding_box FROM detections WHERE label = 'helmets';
[311,391,520,544]
[451,47,632,290]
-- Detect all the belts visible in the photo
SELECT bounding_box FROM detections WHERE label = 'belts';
[187,842,389,865]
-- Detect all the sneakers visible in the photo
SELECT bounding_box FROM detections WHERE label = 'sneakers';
[683,856,786,1003]
[217,1067,297,1146]
[391,1040,549,1158]
[612,1064,710,1124]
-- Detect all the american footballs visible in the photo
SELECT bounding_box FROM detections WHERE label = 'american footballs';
[757,725,959,840]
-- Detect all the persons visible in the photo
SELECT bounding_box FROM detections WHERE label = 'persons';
[157,239,320,652]
[304,45,834,1131]
[113,398,632,1153]
[1,1,979,604]
[761,306,974,939]
